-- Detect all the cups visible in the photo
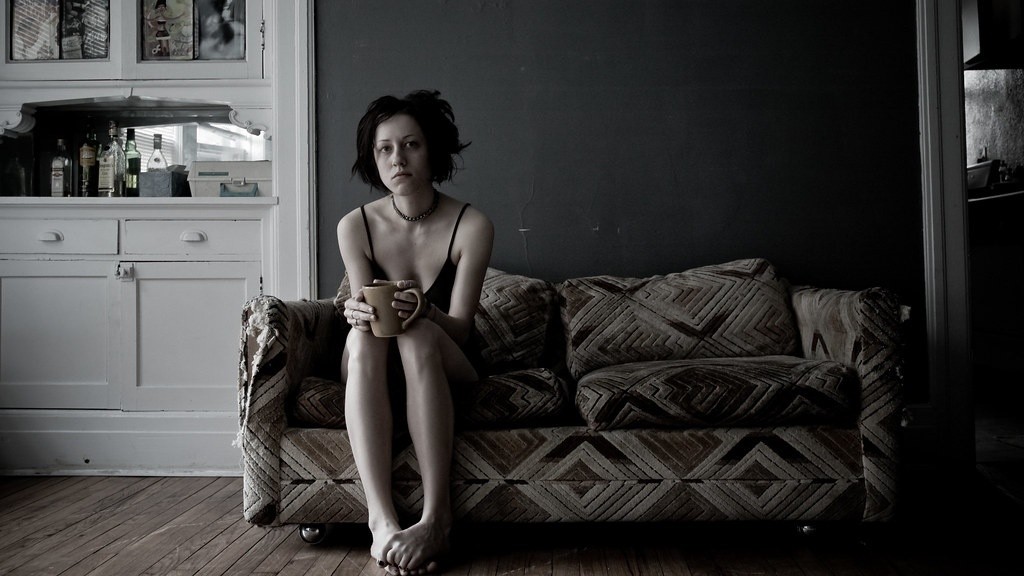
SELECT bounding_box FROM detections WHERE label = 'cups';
[361,280,425,337]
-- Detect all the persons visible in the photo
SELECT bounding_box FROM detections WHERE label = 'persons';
[338,87,495,576]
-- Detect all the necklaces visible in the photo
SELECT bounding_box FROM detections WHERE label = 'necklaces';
[391,187,439,222]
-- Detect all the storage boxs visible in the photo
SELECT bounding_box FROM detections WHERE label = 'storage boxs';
[186,161,273,199]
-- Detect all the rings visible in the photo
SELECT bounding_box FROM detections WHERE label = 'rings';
[351,310,355,319]
[355,319,359,326]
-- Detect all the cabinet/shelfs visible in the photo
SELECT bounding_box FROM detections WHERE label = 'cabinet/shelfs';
[0,0,317,476]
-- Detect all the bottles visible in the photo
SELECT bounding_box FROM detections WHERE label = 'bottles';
[78,124,99,197]
[122,128,142,197]
[146,134,170,197]
[47,138,74,197]
[97,120,127,199]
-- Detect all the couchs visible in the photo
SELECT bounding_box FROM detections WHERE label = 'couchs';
[239,258,909,543]
